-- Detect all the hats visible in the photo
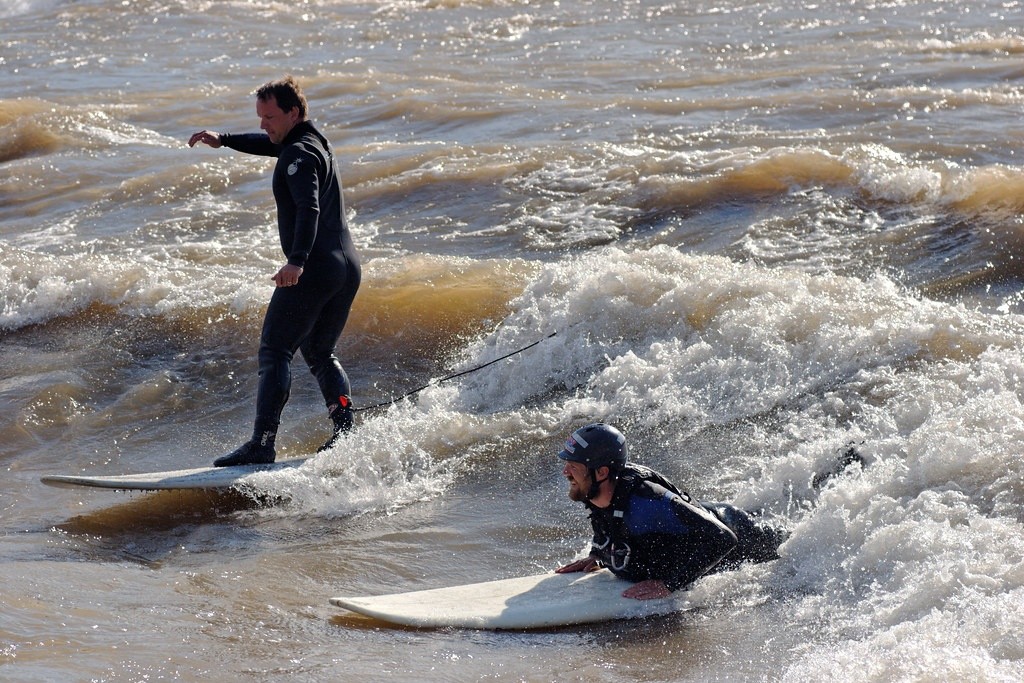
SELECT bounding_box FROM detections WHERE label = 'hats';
[557,423,626,472]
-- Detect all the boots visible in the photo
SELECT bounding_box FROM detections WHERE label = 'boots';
[214,418,280,466]
[318,404,353,453]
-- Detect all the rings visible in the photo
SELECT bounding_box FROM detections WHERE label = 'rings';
[288,282,292,284]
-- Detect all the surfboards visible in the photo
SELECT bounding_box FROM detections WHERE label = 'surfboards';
[326,561,686,632]
[39,454,313,492]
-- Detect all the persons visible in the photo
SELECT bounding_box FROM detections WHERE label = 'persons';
[555,422,867,601]
[189,74,363,465]
